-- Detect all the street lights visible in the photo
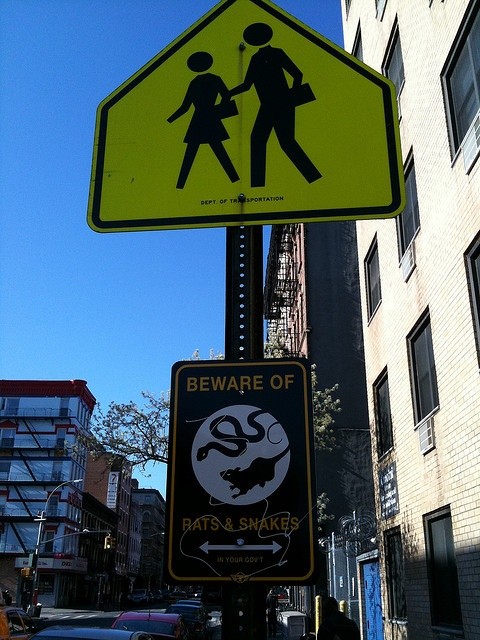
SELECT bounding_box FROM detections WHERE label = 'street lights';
[28,479,84,615]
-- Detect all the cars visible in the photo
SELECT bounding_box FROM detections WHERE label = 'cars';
[30,624,155,640]
[165,603,208,639]
[176,600,203,606]
[167,591,188,604]
[0,607,38,640]
[129,588,150,607]
[110,611,190,640]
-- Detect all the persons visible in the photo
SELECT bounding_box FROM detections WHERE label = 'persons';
[266,587,279,636]
[318,597,360,640]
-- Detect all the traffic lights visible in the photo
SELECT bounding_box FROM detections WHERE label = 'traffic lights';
[106,538,111,548]
[112,538,116,549]
